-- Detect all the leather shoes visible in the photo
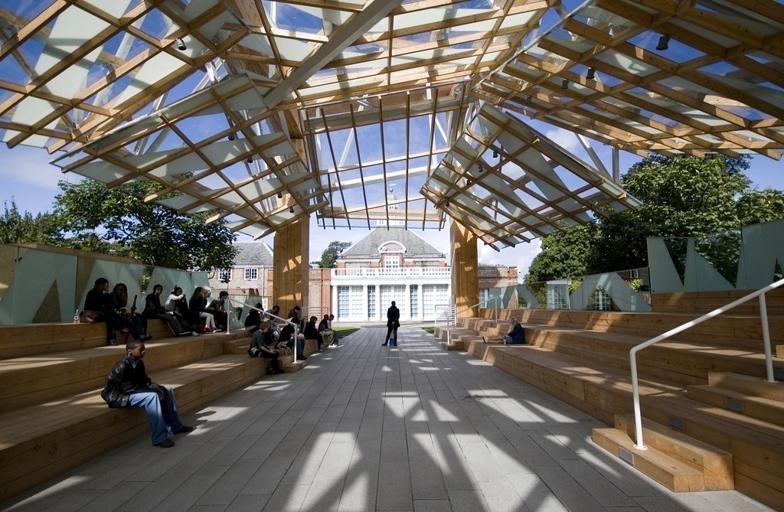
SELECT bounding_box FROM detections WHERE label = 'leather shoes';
[109,334,154,346]
[176,426,194,433]
[152,437,175,448]
[179,325,223,337]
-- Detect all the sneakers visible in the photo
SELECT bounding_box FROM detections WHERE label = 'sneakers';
[297,354,308,360]
[381,342,398,347]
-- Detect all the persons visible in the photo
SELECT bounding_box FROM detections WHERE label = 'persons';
[79,277,339,375]
[382,301,400,346]
[482,317,525,344]
[100,338,194,448]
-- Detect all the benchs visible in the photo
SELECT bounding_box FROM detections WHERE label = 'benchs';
[432,306,784,512]
[0,318,334,497]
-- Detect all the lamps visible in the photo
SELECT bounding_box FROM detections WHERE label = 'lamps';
[655,33,674,51]
[560,66,596,90]
[175,37,295,214]
[466,148,499,186]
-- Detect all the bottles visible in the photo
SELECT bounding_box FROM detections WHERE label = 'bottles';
[74,309,82,322]
[110,328,117,344]
